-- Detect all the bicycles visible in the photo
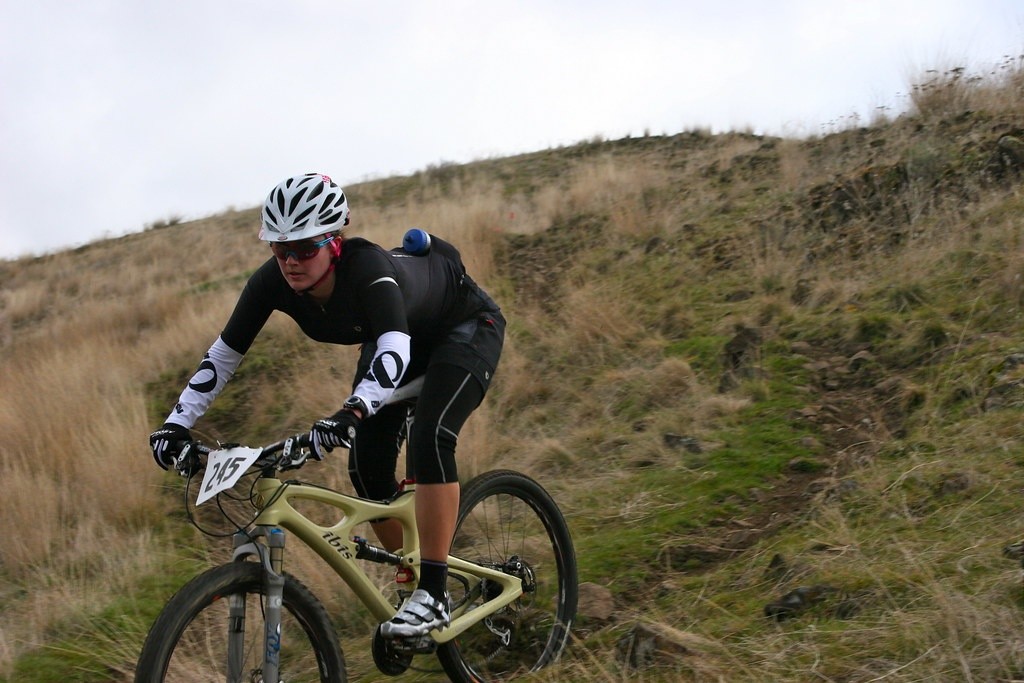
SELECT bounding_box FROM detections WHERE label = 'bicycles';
[135,416,579,683]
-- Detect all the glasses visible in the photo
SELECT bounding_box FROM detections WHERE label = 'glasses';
[269,236,334,261]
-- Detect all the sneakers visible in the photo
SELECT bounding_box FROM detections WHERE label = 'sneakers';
[381,589,450,639]
[389,633,435,655]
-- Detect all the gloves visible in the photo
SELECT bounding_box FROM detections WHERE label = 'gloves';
[152,424,192,471]
[308,409,360,461]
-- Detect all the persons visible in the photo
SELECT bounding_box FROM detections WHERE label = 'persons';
[149,172,507,639]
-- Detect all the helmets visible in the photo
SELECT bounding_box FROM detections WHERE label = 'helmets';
[258,173,350,241]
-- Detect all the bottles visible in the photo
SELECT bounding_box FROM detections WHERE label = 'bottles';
[401,228,432,255]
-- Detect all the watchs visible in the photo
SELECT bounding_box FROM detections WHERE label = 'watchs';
[343,396,368,419]
